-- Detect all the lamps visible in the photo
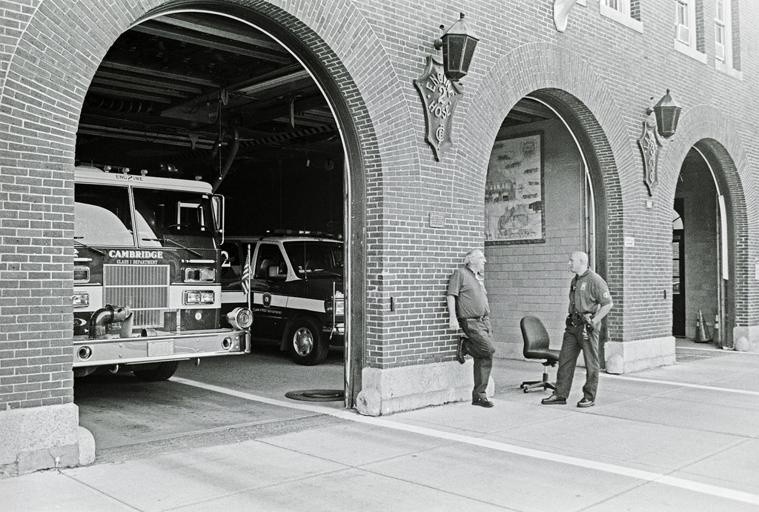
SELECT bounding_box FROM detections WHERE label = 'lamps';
[432,10,481,83]
[645,86,681,138]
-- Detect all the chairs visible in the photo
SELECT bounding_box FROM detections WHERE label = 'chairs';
[519,316,562,394]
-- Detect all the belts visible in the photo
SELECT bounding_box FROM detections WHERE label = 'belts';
[458,315,488,321]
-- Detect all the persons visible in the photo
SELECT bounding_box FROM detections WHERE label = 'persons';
[542,250,614,406]
[446,248,496,408]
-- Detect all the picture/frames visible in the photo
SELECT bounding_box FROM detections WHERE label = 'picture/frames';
[479,130,544,246]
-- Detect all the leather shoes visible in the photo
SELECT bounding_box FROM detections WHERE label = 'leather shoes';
[577,397,595,408]
[542,394,567,405]
[471,398,494,407]
[456,337,467,364]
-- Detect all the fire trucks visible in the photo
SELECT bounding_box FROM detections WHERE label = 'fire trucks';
[220,230,344,364]
[73,164,253,382]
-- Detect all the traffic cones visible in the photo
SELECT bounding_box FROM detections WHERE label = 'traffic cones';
[693,308,712,343]
